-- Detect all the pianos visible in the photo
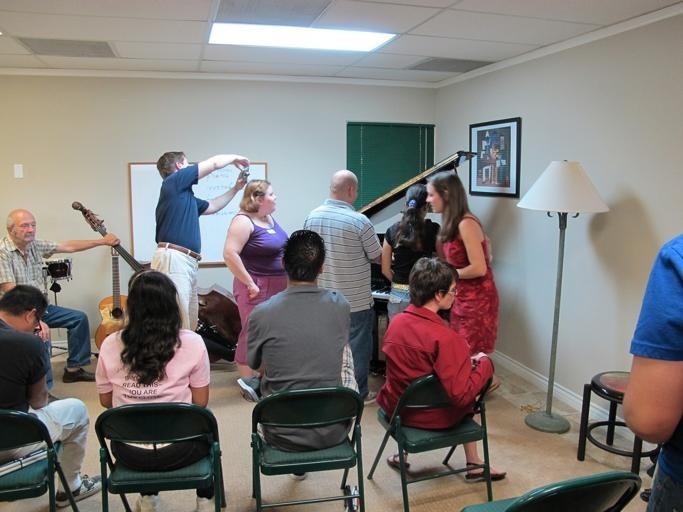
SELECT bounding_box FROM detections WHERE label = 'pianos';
[356,151,477,378]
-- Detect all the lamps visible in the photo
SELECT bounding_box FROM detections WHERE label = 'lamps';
[517,158,610,435]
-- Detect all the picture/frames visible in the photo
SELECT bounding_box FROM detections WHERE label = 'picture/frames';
[468,117,521,199]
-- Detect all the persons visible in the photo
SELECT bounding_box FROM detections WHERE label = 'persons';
[0,209,121,405]
[0,284,102,508]
[479,129,509,186]
[303,168,382,406]
[424,170,502,399]
[95,268,217,512]
[381,183,442,323]
[374,257,508,483]
[621,227,683,512]
[222,179,291,378]
[244,228,360,481]
[149,151,252,332]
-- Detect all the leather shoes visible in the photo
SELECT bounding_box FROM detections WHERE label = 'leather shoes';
[63,368,95,382]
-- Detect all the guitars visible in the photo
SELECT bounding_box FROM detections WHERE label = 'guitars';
[95,246,130,350]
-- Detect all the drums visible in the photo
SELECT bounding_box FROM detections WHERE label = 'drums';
[41,258,71,283]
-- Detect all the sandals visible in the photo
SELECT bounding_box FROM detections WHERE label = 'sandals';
[465,463,506,479]
[387,453,409,470]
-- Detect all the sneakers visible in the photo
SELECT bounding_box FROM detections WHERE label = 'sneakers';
[364,390,378,405]
[237,376,263,402]
[196,496,216,512]
[55,475,102,507]
[136,491,161,512]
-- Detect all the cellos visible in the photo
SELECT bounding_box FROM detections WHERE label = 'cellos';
[72,201,242,364]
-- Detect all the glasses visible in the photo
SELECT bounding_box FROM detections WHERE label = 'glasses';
[33,320,42,334]
[438,288,458,296]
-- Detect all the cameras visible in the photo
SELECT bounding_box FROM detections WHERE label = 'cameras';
[240,167,250,184]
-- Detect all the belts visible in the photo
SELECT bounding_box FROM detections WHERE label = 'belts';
[391,282,410,290]
[158,244,201,261]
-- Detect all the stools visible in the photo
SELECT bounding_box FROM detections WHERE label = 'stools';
[578,371,662,477]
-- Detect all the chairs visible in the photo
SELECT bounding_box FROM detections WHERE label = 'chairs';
[461,470,642,511]
[96,403,226,512]
[367,373,493,511]
[251,387,366,512]
[0,408,82,511]
